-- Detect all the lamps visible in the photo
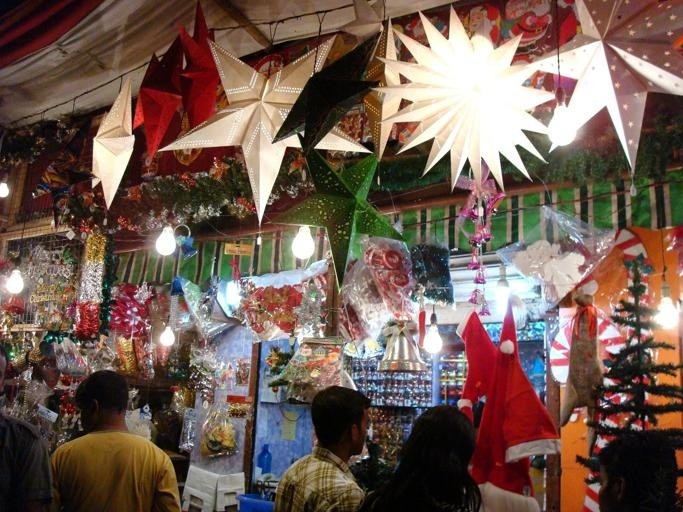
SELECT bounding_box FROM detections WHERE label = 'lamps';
[422,304,443,356]
[155,222,178,258]
[4,215,26,295]
[289,224,316,260]
[547,0,580,148]
[492,263,510,288]
[159,326,176,348]
[0,171,10,198]
[653,184,679,332]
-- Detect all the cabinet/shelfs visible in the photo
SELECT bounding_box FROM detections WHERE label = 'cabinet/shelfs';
[1,218,76,332]
[1,378,189,463]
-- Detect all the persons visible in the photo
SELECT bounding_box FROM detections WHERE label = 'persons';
[1,344,51,511]
[596,440,681,511]
[358,405,486,512]
[49,369,181,511]
[275,384,368,512]
[462,6,497,46]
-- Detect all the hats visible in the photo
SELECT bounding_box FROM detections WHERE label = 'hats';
[499,339,562,465]
